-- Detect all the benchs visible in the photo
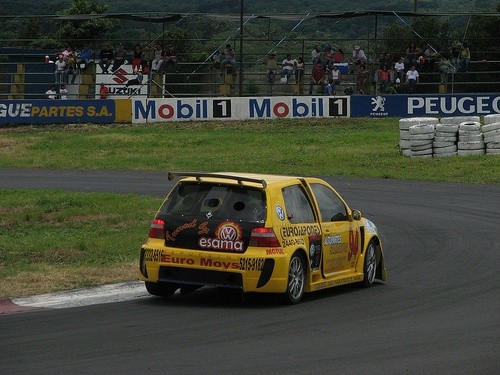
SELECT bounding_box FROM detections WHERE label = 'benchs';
[0,52,500,97]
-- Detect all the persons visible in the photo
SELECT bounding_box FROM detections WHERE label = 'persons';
[220,44,237,76]
[55,43,180,95]
[267,53,304,84]
[308,39,469,95]
[60,84,68,99]
[45,86,57,100]
[100,83,109,99]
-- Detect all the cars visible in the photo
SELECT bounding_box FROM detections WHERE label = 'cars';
[136,170,387,304]
